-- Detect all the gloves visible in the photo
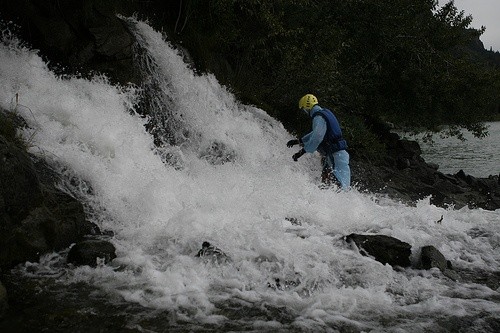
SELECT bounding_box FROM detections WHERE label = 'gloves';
[291,148,305,161]
[286,137,300,148]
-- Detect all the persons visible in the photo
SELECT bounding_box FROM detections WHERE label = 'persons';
[286,94,351,194]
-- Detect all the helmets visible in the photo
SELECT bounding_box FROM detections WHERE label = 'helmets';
[299,94,319,111]
